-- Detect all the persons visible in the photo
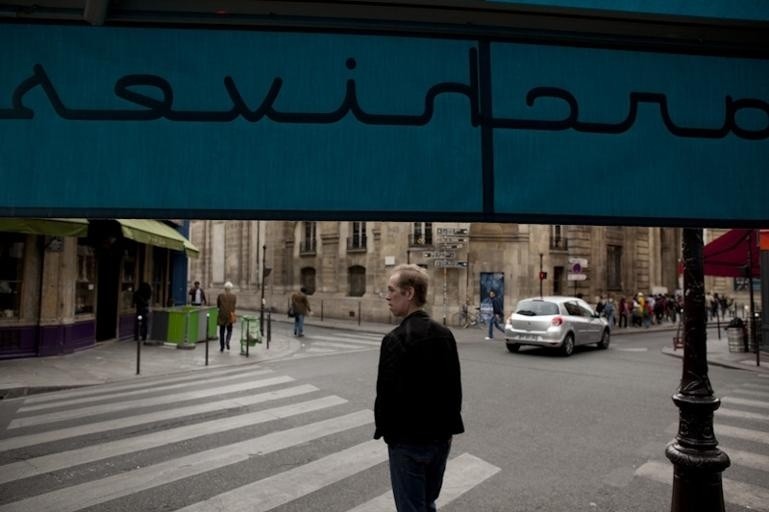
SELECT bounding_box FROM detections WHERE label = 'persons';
[593,290,739,327]
[374,264,465,512]
[482,290,507,341]
[290,287,312,336]
[189,281,207,306]
[217,281,237,352]
[131,282,152,341]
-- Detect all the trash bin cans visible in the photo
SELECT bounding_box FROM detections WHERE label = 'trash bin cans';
[727,327,746,353]
[241,315,262,346]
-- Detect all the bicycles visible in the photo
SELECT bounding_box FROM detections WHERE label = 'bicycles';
[450,301,491,330]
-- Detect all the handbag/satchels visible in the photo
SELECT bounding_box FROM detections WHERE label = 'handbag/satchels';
[288,308,295,317]
[229,312,236,323]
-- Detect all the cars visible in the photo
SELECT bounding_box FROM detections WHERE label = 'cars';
[504,295,613,356]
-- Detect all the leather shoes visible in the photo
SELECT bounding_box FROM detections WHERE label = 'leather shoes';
[294,332,304,337]
[220,343,230,351]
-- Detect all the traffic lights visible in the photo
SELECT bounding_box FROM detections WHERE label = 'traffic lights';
[539,271,549,280]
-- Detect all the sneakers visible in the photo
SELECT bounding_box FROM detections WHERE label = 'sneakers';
[484,336,495,341]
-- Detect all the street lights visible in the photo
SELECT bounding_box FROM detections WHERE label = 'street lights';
[424,228,468,269]
